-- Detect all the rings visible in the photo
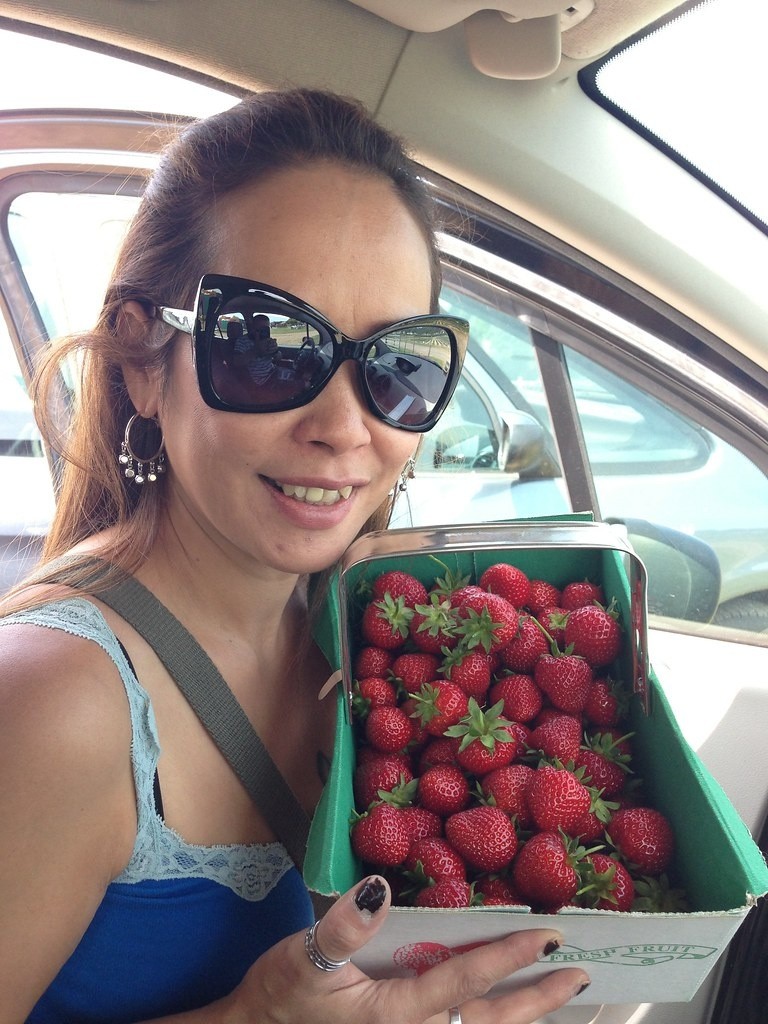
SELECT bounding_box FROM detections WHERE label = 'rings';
[450,1006,462,1024]
[304,921,352,972]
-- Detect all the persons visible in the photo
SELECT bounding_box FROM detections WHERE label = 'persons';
[0,87,592,1024]
[233,315,282,402]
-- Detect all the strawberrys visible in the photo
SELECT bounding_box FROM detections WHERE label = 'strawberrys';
[351,563,674,910]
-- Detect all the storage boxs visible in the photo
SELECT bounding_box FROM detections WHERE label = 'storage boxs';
[303,509,768,1008]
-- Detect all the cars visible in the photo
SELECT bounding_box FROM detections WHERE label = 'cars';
[1,109,768,639]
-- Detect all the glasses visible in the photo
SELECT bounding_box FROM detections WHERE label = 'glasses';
[140,272,471,434]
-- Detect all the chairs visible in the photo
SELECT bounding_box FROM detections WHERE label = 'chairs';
[221,322,297,404]
